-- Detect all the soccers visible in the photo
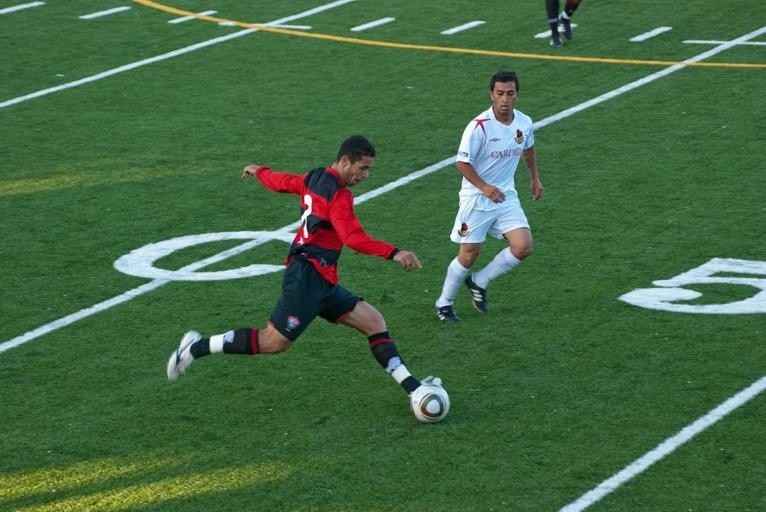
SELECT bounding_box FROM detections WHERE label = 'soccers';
[412,384,450,422]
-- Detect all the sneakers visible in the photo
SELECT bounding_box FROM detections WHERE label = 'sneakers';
[165,328,204,382]
[558,13,573,39]
[465,271,489,315]
[433,299,461,322]
[551,33,561,47]
[408,375,445,413]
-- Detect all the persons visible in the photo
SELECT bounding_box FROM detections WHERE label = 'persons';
[544,0,582,49]
[166,134,443,398]
[434,72,545,323]
